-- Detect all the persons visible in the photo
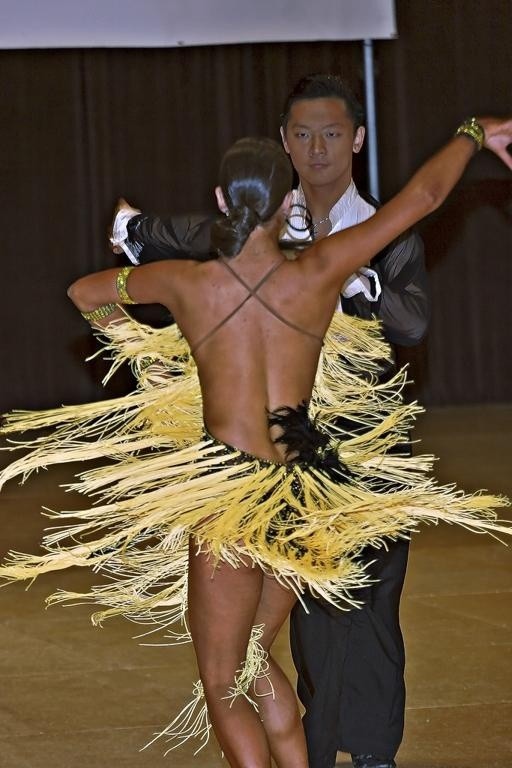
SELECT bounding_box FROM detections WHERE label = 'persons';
[107,73,436,768]
[64,110,510,768]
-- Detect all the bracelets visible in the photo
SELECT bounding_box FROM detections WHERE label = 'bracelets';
[455,112,487,154]
[80,303,114,326]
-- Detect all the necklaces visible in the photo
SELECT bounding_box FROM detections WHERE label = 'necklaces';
[304,210,331,234]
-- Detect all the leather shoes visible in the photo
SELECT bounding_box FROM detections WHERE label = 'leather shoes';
[309,751,400,767]
